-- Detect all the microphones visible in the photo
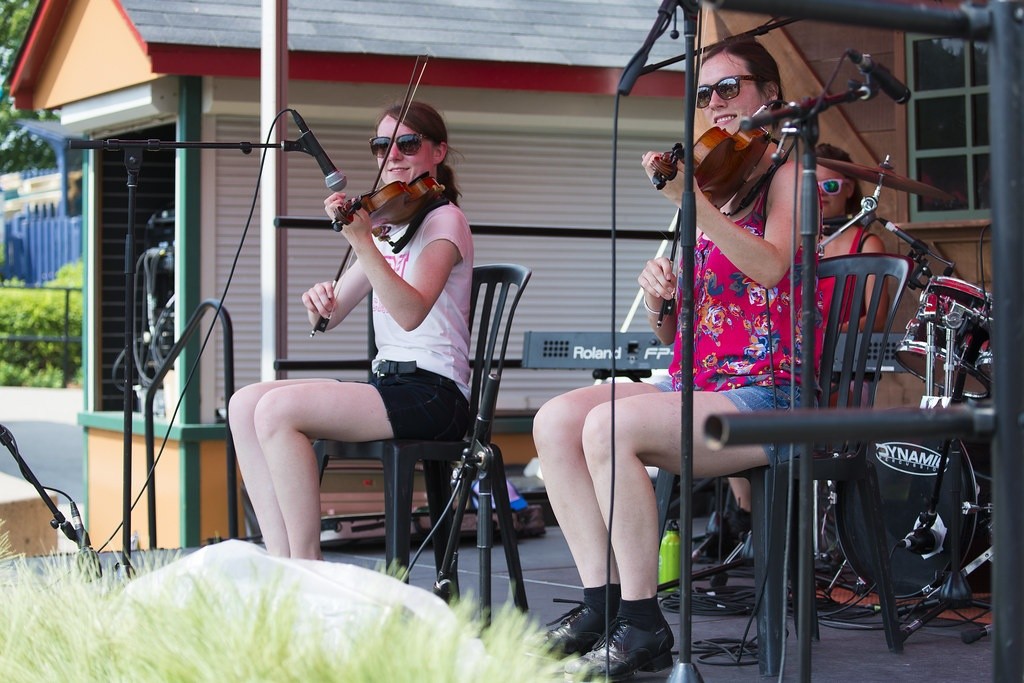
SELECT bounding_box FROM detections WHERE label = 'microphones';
[846,48,912,105]
[896,528,942,555]
[70,502,101,583]
[292,109,346,192]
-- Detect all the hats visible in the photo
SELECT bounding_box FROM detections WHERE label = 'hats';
[468,475,527,511]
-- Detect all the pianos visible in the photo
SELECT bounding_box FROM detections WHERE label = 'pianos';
[522,330,910,553]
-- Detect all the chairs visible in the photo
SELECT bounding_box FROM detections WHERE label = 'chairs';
[311,259,536,630]
[654,248,910,683]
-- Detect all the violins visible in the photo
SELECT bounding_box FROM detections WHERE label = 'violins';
[650,122,771,210]
[333,175,446,226]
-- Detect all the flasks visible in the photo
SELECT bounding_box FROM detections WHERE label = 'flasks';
[657,520,680,594]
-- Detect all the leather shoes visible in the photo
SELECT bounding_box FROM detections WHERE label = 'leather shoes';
[563,611,675,682]
[538,598,612,655]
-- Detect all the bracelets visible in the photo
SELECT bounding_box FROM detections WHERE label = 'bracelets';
[642,297,668,318]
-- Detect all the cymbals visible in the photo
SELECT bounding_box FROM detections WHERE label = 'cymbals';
[815,155,957,201]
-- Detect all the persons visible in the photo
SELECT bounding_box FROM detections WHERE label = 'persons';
[229,101,472,560]
[533,34,818,683]
[691,141,889,561]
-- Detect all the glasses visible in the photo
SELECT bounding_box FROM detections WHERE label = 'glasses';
[369,133,425,158]
[696,74,759,108]
[819,179,851,195]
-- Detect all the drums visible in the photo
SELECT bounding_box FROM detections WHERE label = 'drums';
[892,274,994,401]
